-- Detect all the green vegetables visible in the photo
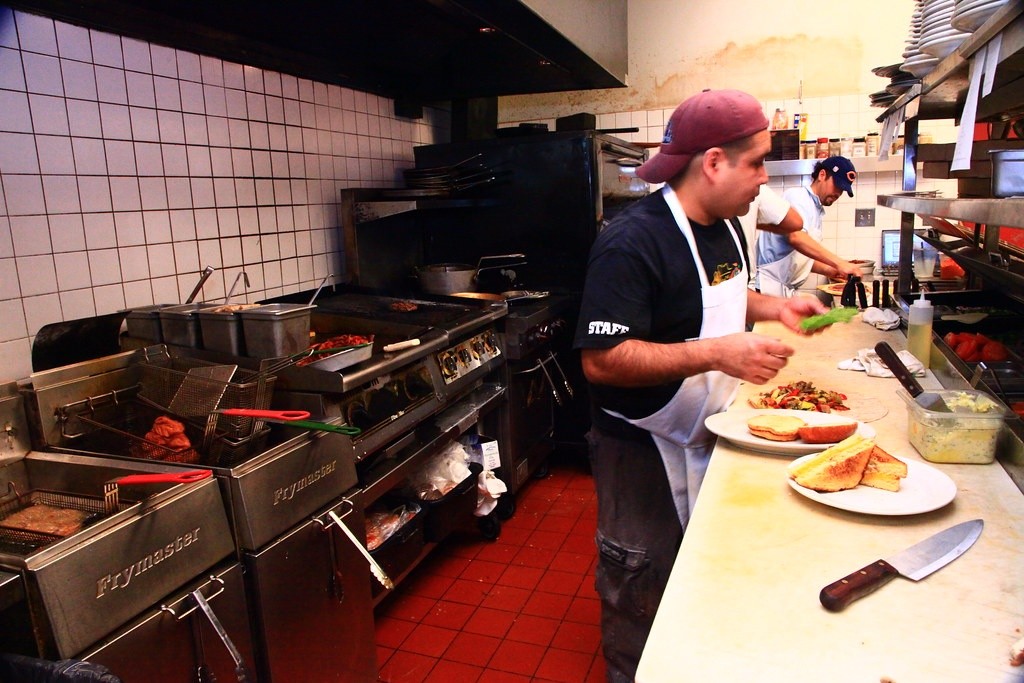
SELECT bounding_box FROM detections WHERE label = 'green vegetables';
[801,306,860,331]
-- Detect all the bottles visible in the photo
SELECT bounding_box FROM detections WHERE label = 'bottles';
[794,100,808,139]
[908,289,933,369]
[866,158,868,163]
[772,106,788,130]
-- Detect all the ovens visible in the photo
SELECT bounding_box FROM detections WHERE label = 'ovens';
[414,128,650,468]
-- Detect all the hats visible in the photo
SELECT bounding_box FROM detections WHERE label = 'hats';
[821,155,856,198]
[636,83,770,184]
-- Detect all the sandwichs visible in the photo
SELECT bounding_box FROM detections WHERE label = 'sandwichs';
[791,433,908,492]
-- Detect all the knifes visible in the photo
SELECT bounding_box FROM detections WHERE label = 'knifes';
[841,273,898,307]
[819,519,984,612]
[875,342,951,413]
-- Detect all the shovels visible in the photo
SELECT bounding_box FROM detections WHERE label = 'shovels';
[873,341,952,413]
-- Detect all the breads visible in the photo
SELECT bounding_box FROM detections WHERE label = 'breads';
[943,332,1006,362]
[747,414,858,444]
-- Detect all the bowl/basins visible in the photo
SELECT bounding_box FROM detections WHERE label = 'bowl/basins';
[849,260,876,274]
[894,389,1021,465]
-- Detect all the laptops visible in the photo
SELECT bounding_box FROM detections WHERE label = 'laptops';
[881,229,938,274]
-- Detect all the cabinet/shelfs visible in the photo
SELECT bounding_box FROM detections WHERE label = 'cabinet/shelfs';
[876,0,1024,496]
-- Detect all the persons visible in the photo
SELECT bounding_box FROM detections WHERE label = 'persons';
[737,183,803,290]
[573,89,833,683]
[756,156,863,298]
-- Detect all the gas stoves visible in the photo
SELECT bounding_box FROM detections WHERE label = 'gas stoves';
[253,283,509,460]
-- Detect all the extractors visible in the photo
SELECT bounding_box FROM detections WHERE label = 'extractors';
[0,0,628,119]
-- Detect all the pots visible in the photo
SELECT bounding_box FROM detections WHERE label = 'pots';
[385,151,496,200]
[409,253,529,301]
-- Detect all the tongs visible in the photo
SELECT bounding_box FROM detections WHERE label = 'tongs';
[537,358,562,407]
[549,351,574,398]
[188,588,253,683]
[326,510,395,605]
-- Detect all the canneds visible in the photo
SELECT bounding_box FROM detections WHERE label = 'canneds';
[800,132,904,160]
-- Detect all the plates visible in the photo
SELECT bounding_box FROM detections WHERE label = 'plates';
[500,290,550,301]
[703,409,881,457]
[786,452,957,513]
[869,0,1009,107]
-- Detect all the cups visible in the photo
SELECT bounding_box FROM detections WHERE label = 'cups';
[914,249,937,279]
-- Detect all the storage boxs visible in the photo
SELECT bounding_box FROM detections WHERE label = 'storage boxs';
[381,462,485,544]
[765,129,800,161]
[475,433,501,470]
[366,496,425,597]
